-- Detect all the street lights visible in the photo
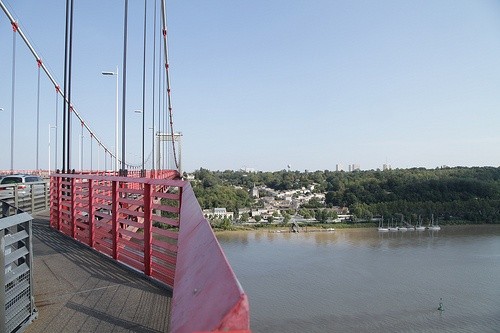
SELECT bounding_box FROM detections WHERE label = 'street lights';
[47,123,54,174]
[134,110,143,170]
[102,65,119,175]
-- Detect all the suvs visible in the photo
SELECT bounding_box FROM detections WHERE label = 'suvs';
[0,174,49,202]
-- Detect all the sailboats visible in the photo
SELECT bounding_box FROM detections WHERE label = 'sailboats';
[376,214,442,232]
